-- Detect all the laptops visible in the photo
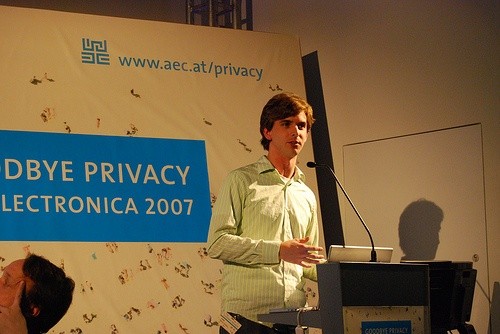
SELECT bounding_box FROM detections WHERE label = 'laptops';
[269,245,393,314]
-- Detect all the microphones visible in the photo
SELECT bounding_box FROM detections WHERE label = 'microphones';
[307,161,379,262]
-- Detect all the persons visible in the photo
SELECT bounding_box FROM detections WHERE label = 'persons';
[207,92,327,334]
[0,253,75,334]
